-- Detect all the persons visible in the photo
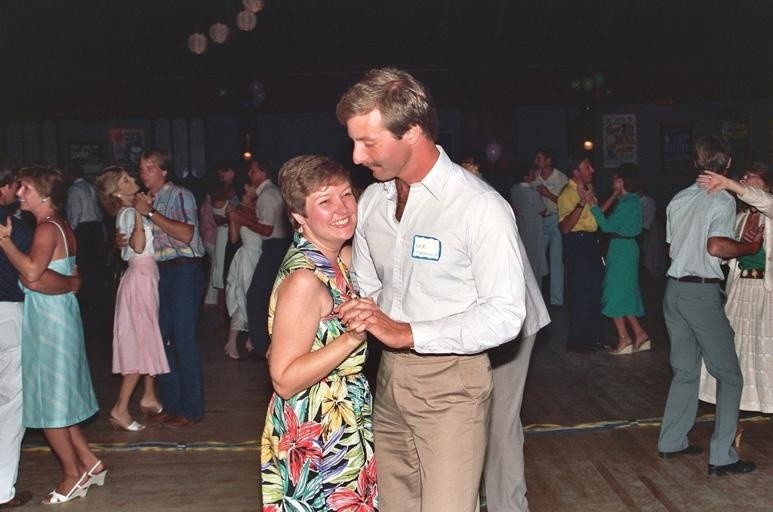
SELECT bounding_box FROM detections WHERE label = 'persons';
[260,154,380,510]
[640,187,658,240]
[0,157,82,508]
[224,158,285,360]
[511,163,549,291]
[96,166,164,433]
[458,159,552,511]
[657,133,765,478]
[558,152,619,356]
[637,188,657,229]
[511,146,569,312]
[66,165,106,232]
[583,162,652,356]
[114,147,207,432]
[641,224,664,276]
[224,172,262,360]
[337,65,526,512]
[696,167,773,448]
[266,69,377,512]
[201,183,235,309]
[461,147,490,183]
[0,166,108,505]
[201,159,242,311]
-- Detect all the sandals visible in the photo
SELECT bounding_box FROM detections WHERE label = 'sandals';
[633,335,652,352]
[609,336,632,356]
[42,470,92,505]
[88,461,107,486]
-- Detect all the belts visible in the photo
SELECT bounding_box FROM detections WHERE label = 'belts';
[158,256,202,266]
[669,275,720,284]
[740,270,764,278]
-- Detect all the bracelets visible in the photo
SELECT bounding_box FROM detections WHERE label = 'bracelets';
[0,235,12,242]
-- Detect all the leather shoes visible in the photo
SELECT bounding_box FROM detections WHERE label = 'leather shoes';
[709,460,757,478]
[566,347,596,358]
[658,446,702,461]
[147,409,174,423]
[164,415,196,431]
[0,491,33,509]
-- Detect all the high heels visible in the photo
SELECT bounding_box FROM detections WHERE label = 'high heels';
[735,423,742,447]
[224,344,240,362]
[109,417,146,434]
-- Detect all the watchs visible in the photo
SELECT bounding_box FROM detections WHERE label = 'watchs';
[577,203,584,209]
[147,209,156,220]
